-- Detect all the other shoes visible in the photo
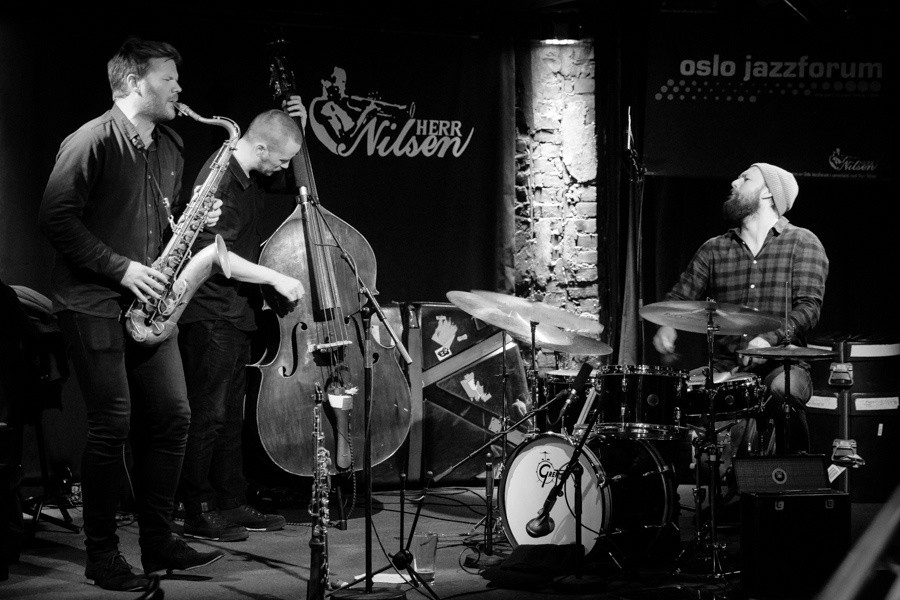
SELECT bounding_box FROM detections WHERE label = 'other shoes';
[173,502,184,525]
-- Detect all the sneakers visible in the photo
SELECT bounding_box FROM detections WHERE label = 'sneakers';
[184,510,248,541]
[219,504,286,531]
[85,550,144,592]
[141,538,224,573]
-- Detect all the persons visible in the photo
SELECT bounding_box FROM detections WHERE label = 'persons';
[653,163,830,459]
[38,40,224,592]
[177,95,307,542]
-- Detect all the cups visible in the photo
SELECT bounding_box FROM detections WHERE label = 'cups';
[411,531,438,584]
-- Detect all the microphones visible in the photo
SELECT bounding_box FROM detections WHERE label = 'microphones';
[562,362,593,413]
[525,514,556,539]
[299,186,308,212]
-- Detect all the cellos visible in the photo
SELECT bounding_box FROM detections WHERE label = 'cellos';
[255,29,413,478]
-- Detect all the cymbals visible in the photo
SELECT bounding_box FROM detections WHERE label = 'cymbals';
[447,289,839,362]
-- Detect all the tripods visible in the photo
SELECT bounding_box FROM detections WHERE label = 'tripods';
[675,309,738,584]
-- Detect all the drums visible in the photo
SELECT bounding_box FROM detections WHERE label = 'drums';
[498,430,669,556]
[598,364,679,427]
[532,368,604,433]
[684,372,763,413]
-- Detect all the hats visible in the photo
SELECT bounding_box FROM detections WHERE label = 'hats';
[751,163,799,217]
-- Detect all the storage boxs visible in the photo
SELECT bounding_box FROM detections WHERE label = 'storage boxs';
[370,298,536,492]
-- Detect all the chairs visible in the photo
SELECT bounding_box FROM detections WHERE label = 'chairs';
[0,282,74,529]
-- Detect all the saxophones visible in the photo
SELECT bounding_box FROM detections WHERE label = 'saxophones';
[123,101,241,347]
[307,382,331,600]
